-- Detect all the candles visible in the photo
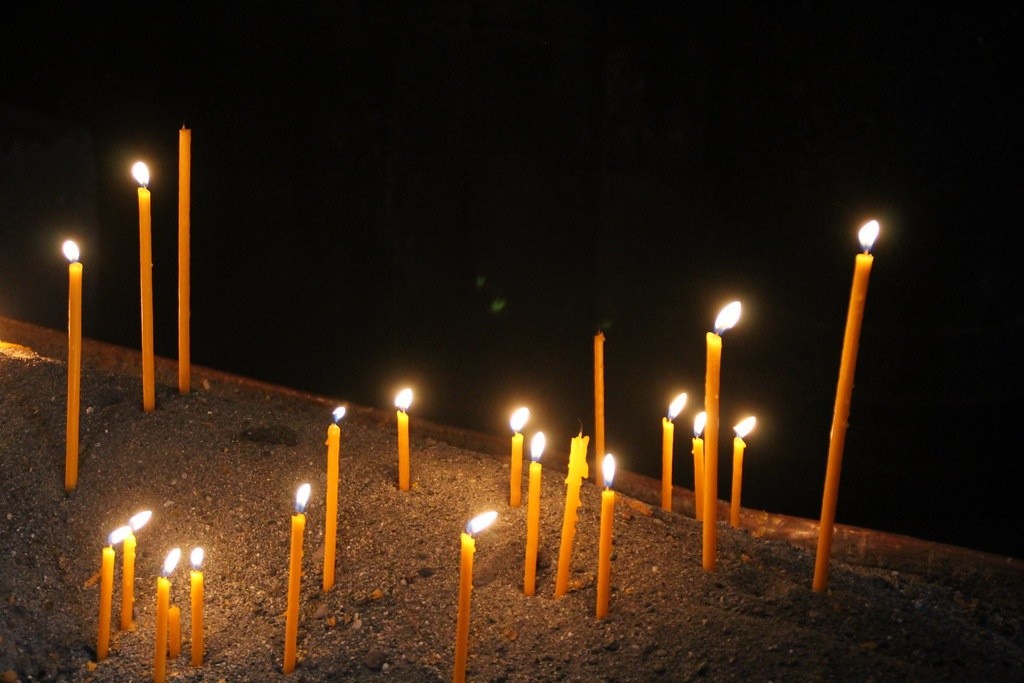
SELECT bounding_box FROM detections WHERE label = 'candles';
[453,531,476,683]
[814,252,874,590]
[97,546,115,660]
[691,438,705,520]
[730,436,747,528]
[704,331,723,575]
[66,262,84,492]
[323,422,341,592]
[397,410,410,491]
[138,188,155,409]
[524,462,543,595]
[597,489,616,618]
[283,514,306,674]
[556,435,591,597]
[595,331,606,486]
[510,433,524,506]
[178,127,191,393]
[120,532,136,632]
[170,606,182,659]
[153,576,173,683]
[190,569,204,665]
[661,416,673,513]
